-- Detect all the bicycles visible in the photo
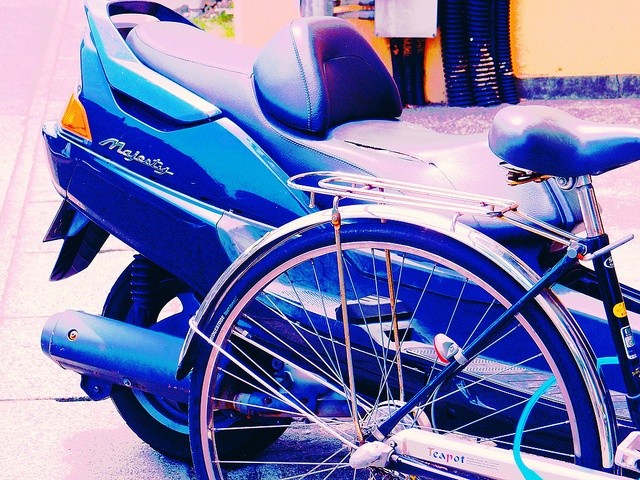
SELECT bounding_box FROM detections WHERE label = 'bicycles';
[177,106,639,480]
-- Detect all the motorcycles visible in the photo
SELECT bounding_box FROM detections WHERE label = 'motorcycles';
[41,0,640,480]
